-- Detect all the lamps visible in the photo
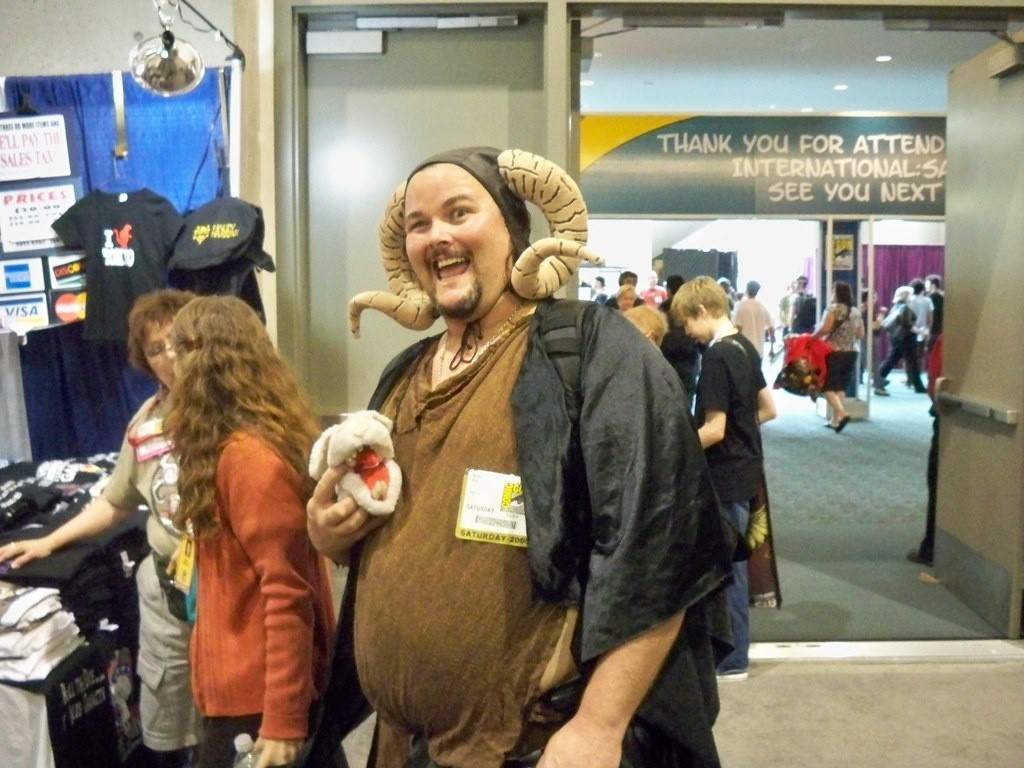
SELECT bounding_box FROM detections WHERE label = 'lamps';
[128,1,247,96]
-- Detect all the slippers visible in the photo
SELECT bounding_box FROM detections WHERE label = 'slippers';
[825,423,836,429]
[835,415,851,433]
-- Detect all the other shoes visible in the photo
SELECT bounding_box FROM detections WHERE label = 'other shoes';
[922,388,928,392]
[874,389,890,395]
[873,377,889,387]
[907,552,934,566]
[715,667,748,682]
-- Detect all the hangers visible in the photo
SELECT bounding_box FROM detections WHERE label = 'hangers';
[98,150,150,193]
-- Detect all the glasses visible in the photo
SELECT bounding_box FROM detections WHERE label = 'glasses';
[141,332,174,359]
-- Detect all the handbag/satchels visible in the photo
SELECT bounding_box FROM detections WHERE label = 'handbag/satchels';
[891,306,909,350]
[773,332,833,402]
[152,550,189,621]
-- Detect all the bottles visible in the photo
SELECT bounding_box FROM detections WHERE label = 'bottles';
[233,733,261,768]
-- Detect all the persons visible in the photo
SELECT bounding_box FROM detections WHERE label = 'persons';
[592,271,944,681]
[308,147,722,768]
[1,290,337,768]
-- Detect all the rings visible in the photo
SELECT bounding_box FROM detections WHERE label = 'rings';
[10,542,16,551]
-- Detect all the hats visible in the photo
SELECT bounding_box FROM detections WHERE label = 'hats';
[892,286,914,303]
[717,277,734,292]
[349,147,604,338]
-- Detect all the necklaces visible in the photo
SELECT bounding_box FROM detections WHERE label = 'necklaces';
[438,301,524,385]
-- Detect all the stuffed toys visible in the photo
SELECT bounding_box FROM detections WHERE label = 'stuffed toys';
[308,410,402,515]
